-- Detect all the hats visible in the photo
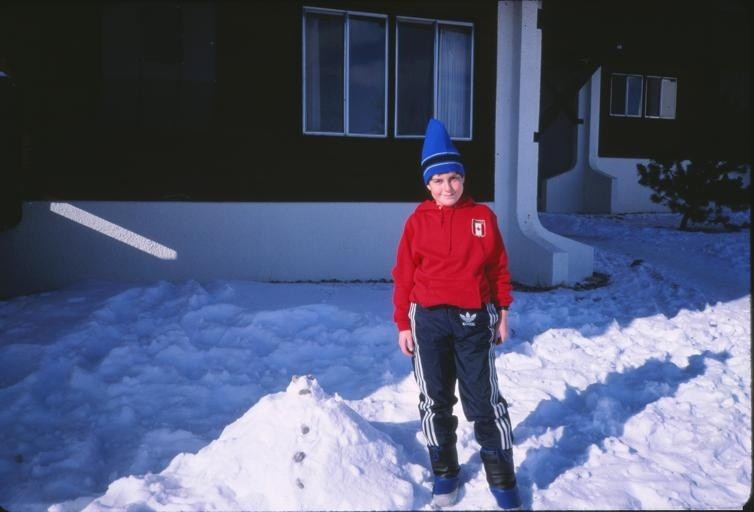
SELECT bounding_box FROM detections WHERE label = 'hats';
[420,118,464,186]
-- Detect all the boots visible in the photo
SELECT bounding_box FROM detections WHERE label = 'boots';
[426,442,461,507]
[479,447,523,511]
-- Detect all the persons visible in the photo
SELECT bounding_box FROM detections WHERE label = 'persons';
[392,119,524,511]
